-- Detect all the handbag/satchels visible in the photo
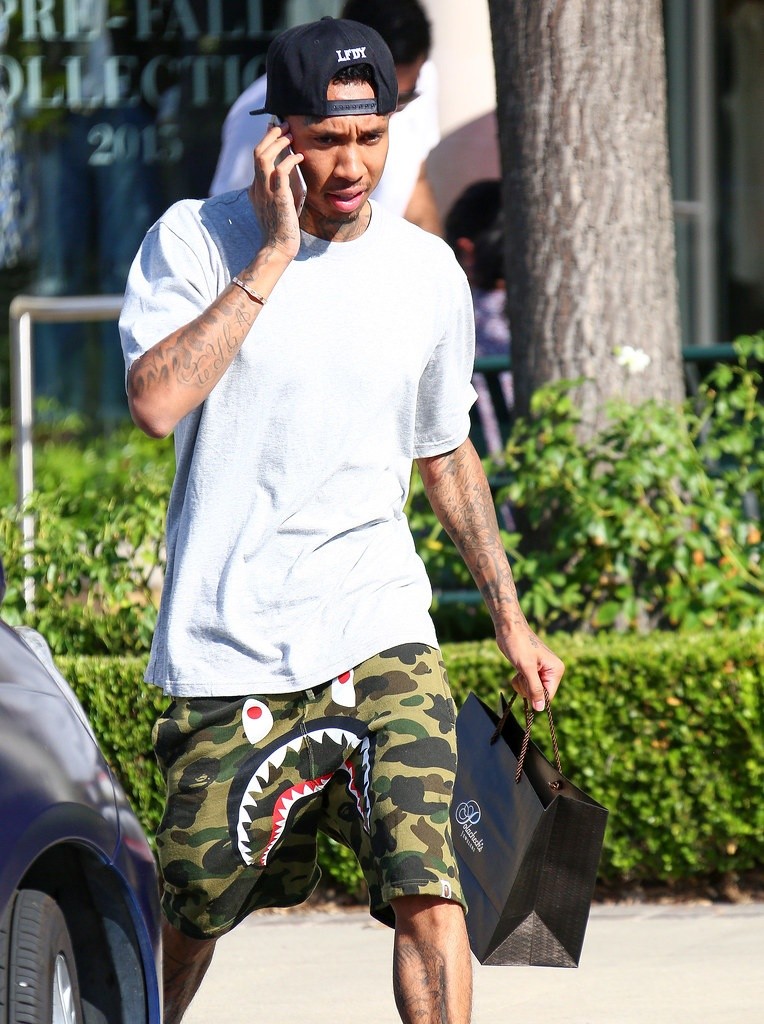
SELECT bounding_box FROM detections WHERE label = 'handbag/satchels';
[446,675,609,969]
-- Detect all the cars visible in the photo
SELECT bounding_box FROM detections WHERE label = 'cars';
[1,617,168,1024]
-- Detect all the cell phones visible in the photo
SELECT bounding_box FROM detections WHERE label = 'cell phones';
[266,115,308,217]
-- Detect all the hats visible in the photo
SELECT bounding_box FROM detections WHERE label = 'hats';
[250,17,398,116]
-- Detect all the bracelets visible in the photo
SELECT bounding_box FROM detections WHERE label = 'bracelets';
[232,275,268,306]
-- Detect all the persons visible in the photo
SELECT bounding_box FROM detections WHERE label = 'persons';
[437,179,522,533]
[112,17,571,1022]
[204,1,445,236]
